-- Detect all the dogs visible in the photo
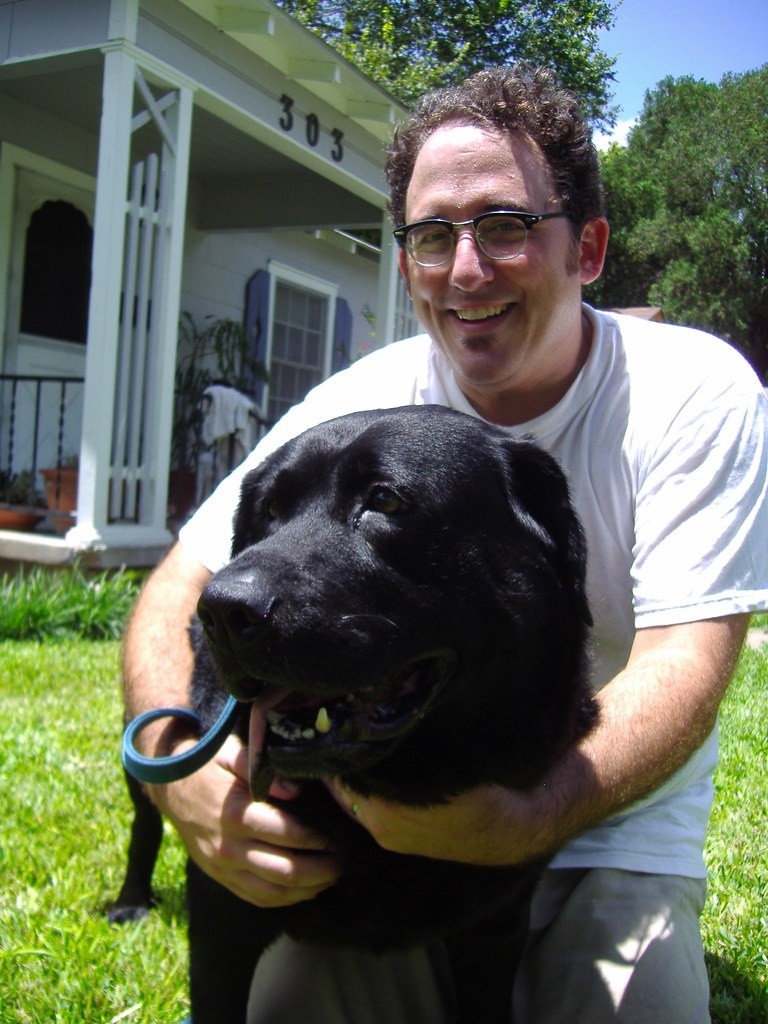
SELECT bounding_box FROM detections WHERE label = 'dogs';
[97,405,600,1024]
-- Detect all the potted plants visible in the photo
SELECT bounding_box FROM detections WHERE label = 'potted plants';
[166,308,220,518]
[39,454,78,535]
[0,452,47,536]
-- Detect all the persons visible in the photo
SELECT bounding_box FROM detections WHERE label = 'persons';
[121,58,768,1024]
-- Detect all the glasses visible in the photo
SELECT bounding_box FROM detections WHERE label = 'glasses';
[393,211,568,267]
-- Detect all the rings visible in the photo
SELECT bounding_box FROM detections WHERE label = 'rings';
[352,803,358,815]
